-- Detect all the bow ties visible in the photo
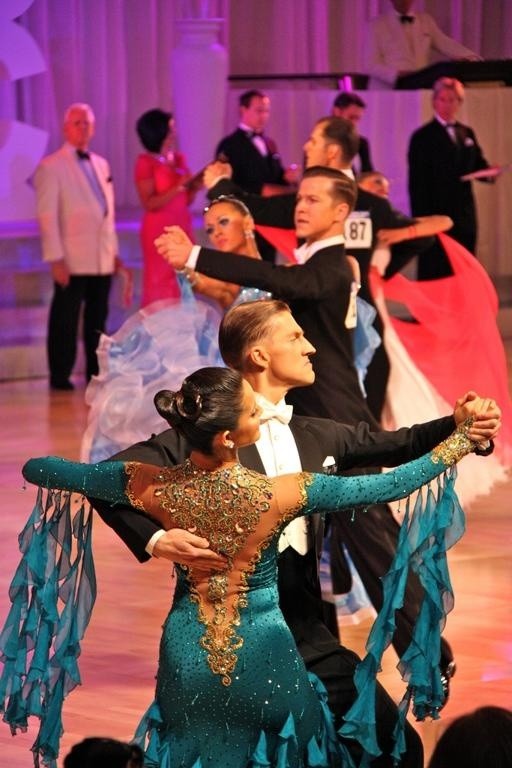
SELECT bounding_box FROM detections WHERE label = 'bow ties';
[249,131,264,138]
[76,148,90,160]
[445,122,458,128]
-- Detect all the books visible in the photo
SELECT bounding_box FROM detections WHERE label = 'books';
[461,164,502,184]
[181,156,230,190]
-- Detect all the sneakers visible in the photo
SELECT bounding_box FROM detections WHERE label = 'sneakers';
[50,377,75,390]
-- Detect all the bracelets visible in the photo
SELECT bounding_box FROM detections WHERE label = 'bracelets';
[180,267,200,290]
[409,226,419,240]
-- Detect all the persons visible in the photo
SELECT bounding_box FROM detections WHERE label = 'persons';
[61,737,158,768]
[206,114,455,283]
[427,705,511,768]
[407,77,504,281]
[154,166,456,718]
[134,108,205,321]
[84,298,502,768]
[230,165,512,524]
[303,92,374,183]
[34,102,122,391]
[78,195,378,631]
[217,88,289,264]
[0,367,490,768]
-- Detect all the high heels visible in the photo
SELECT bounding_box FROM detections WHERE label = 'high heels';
[427,658,455,719]
